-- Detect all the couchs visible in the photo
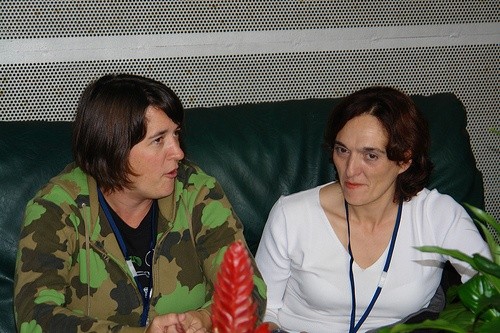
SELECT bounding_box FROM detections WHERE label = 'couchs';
[0,92,488,333]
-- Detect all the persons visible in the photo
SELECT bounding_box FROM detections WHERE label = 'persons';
[253,87,495,333]
[12,73,266,333]
